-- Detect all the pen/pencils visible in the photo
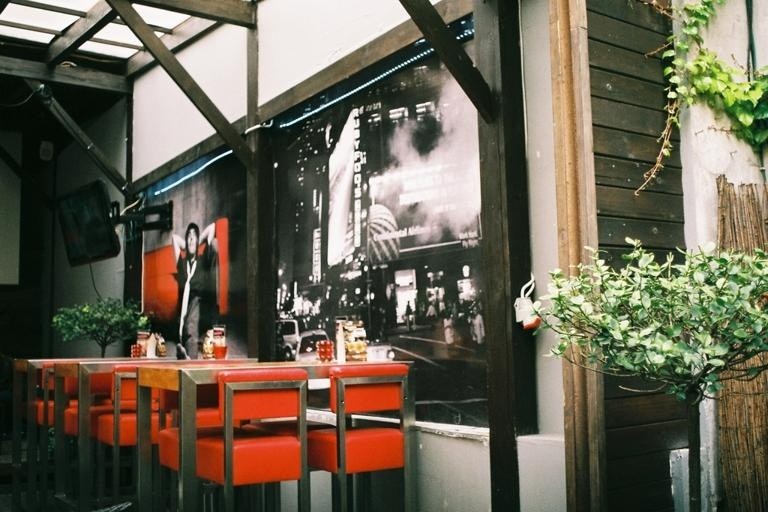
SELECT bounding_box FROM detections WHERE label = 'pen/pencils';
[277,318,299,358]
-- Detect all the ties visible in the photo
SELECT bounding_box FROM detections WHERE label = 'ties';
[56,177,120,267]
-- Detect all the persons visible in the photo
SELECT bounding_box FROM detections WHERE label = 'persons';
[405,295,487,360]
[171,221,222,362]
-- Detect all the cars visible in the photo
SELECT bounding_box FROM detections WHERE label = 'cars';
[318,342,333,362]
[211,324,226,346]
[213,346,228,359]
[130,345,142,358]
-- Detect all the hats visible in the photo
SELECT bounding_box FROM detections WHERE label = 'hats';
[292,328,331,362]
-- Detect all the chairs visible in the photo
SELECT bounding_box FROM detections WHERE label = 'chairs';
[8,349,424,512]
